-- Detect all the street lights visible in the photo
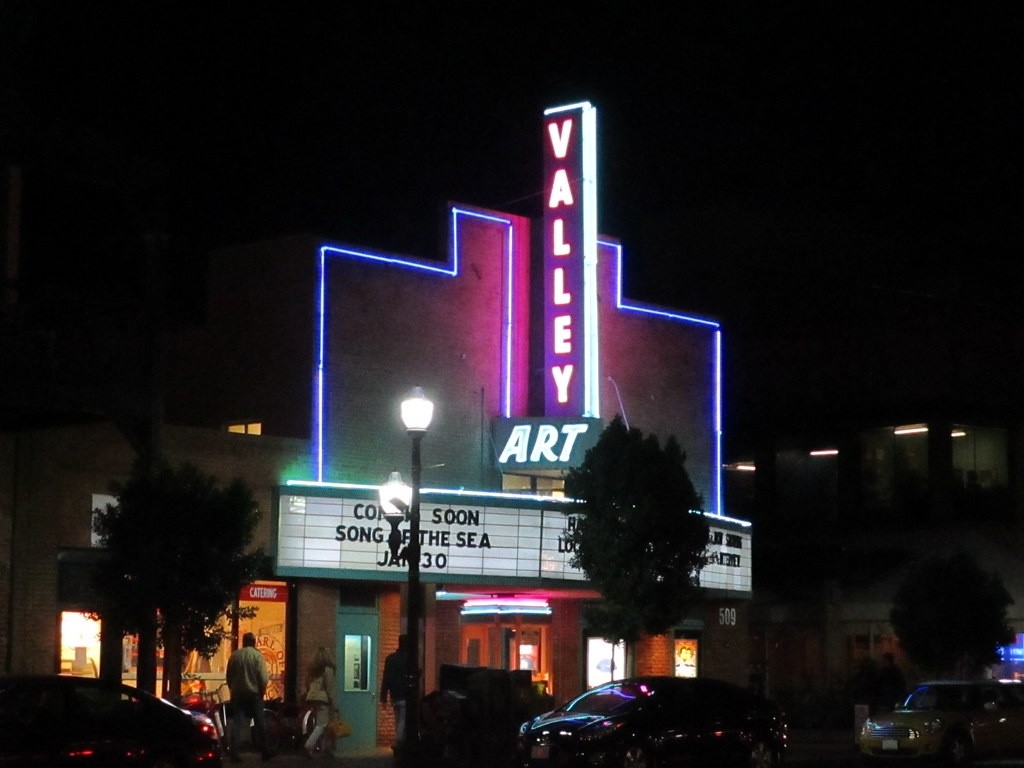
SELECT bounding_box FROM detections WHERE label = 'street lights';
[378,384,434,768]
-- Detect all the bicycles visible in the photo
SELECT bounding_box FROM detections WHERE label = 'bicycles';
[196,682,231,755]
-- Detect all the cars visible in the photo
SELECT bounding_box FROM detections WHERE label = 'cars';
[0,674,223,767]
[519,675,787,768]
[856,679,1024,768]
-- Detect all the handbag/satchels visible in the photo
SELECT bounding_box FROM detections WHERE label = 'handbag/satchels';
[327,709,352,738]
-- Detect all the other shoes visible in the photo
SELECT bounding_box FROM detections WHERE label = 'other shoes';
[304,744,312,758]
[263,751,271,762]
[391,743,399,757]
[231,755,242,763]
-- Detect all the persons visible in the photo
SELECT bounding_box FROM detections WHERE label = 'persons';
[381,635,422,755]
[848,652,906,751]
[226,632,276,762]
[304,646,339,758]
